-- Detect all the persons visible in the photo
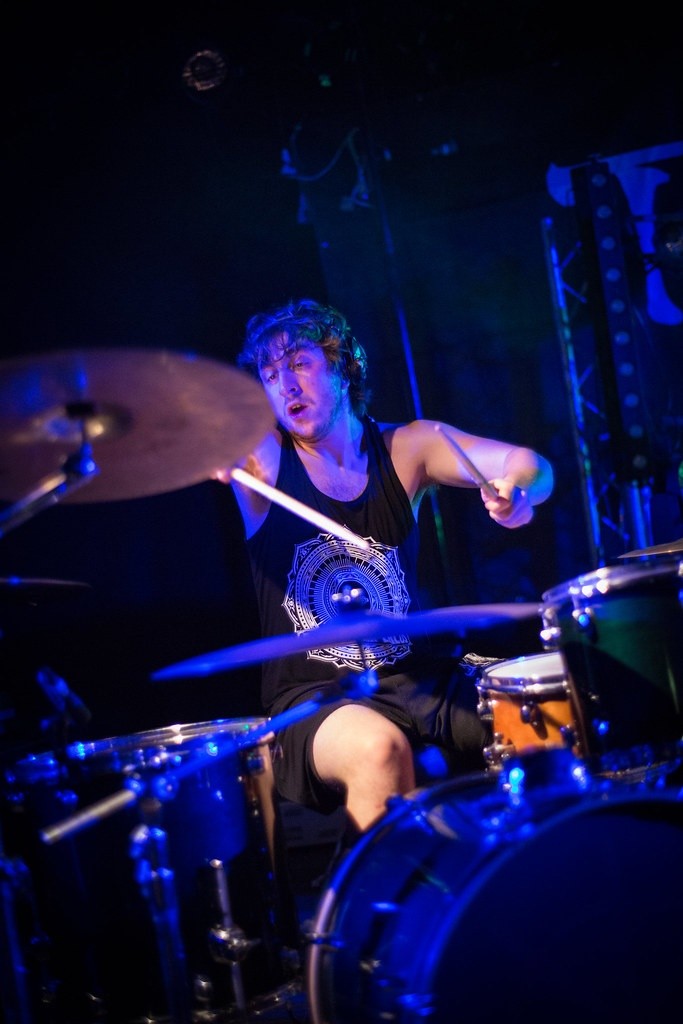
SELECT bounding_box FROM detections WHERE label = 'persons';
[209,300,555,831]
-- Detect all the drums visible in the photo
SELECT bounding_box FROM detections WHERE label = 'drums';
[539,559,683,783]
[301,758,683,1023]
[2,714,283,1023]
[478,651,591,772]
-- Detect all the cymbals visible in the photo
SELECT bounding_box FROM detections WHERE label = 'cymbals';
[0,341,282,507]
[148,600,546,694]
[0,411,91,538]
[2,565,90,595]
[615,538,682,561]
